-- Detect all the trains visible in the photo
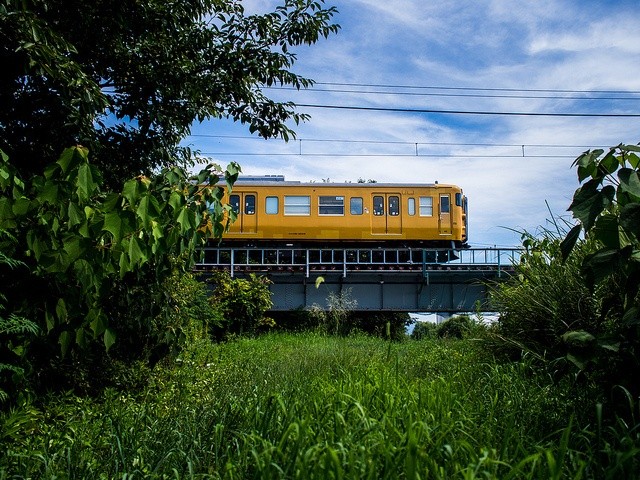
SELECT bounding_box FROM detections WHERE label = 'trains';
[180,175,472,267]
